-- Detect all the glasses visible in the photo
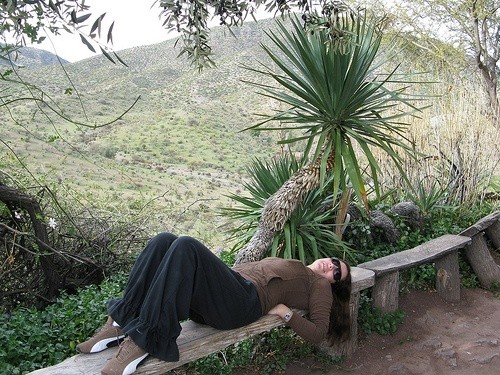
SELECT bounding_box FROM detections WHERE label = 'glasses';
[332,257,341,284]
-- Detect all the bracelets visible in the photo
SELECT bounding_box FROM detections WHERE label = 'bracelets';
[283,311,294,321]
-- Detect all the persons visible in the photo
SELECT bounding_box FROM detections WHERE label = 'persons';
[75,231,357,375]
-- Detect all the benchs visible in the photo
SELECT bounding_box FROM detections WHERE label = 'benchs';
[357,234,471,312]
[457,210,500,287]
[26,265,375,375]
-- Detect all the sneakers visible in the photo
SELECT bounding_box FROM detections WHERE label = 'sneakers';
[76,315,128,354]
[101,335,150,375]
